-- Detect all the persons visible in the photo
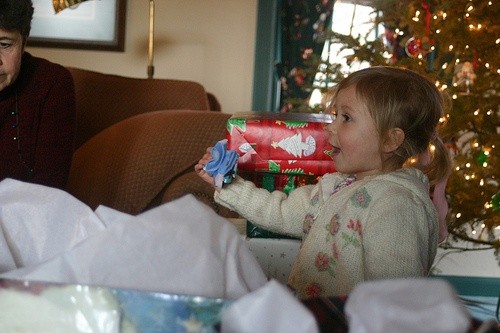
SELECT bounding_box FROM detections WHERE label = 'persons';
[0,0,77,196]
[195,66,453,300]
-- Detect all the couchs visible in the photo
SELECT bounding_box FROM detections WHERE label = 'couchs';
[65,67,241,218]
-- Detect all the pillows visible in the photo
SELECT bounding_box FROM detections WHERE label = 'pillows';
[73,110,234,216]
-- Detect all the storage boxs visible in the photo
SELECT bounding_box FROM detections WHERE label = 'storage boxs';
[226,112,337,238]
[226,219,302,285]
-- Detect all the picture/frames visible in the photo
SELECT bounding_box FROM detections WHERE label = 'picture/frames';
[25,0,126,52]
